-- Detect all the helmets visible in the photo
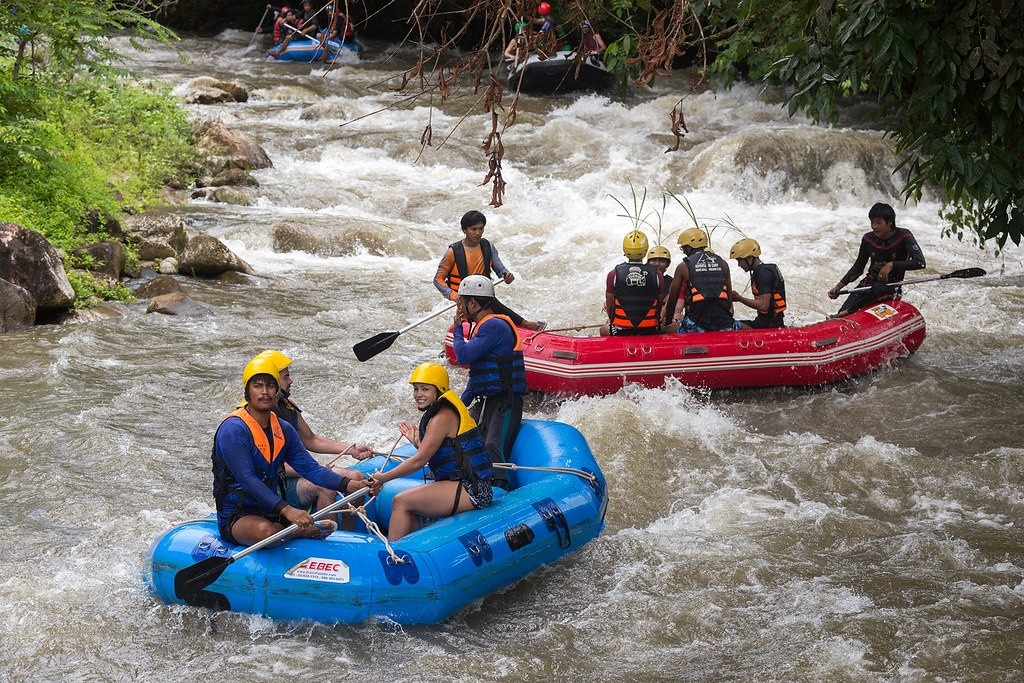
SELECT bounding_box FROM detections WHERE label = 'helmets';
[243,359,280,389]
[677,228,708,248]
[704,247,715,254]
[253,350,292,372]
[409,362,449,393]
[730,238,761,260]
[458,275,495,297]
[648,246,671,268]
[623,230,648,260]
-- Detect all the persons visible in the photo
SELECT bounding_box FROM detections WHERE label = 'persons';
[728,238,787,330]
[503,2,607,69]
[369,362,495,542]
[600,227,743,336]
[828,203,926,316]
[452,275,530,491]
[433,211,548,332]
[211,350,382,550]
[255,0,354,64]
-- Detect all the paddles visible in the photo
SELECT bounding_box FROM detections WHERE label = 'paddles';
[284,22,321,43]
[173,475,376,599]
[353,271,506,362]
[836,268,986,295]
[482,57,516,70]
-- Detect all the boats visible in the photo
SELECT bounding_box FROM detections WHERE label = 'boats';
[443,299,927,396]
[269,30,360,61]
[149,413,611,625]
[507,50,613,95]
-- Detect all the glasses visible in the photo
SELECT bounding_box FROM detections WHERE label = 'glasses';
[680,246,684,251]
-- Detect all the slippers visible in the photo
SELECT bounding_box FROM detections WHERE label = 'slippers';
[532,321,548,332]
[302,519,338,540]
